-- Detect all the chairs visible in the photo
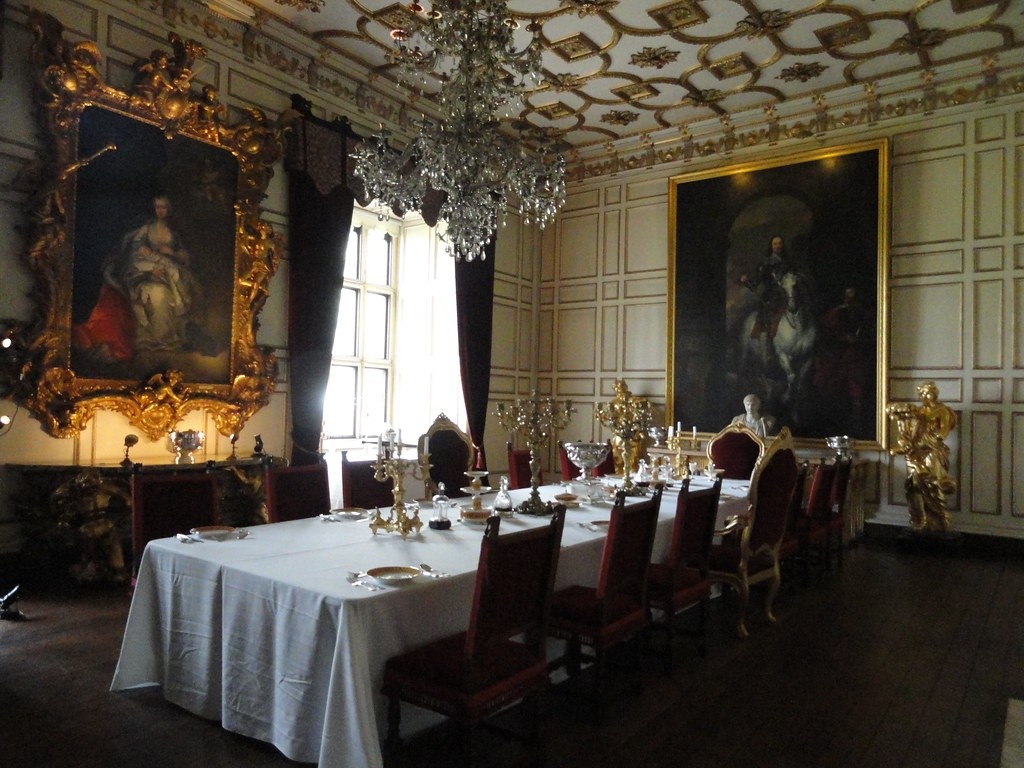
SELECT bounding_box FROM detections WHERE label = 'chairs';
[649,472,725,668]
[711,427,801,639]
[343,462,395,510]
[263,462,330,524]
[558,439,583,482]
[706,420,765,481]
[802,457,826,577]
[129,469,219,588]
[827,453,854,570]
[783,460,802,586]
[507,442,544,490]
[545,481,666,726]
[383,502,567,768]
[590,438,615,477]
[417,413,480,501]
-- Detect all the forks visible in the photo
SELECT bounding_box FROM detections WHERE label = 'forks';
[345,575,376,591]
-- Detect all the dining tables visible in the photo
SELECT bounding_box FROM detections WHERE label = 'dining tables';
[110,469,759,768]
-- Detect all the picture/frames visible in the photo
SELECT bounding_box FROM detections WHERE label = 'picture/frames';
[0,3,296,443]
[663,137,891,450]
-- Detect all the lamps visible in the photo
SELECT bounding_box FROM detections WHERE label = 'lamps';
[343,0,568,264]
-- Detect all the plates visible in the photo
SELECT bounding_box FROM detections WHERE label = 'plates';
[189,525,235,541]
[366,566,421,586]
[329,508,369,520]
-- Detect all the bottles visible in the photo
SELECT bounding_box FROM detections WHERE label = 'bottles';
[493,475,513,519]
[429,482,451,530]
